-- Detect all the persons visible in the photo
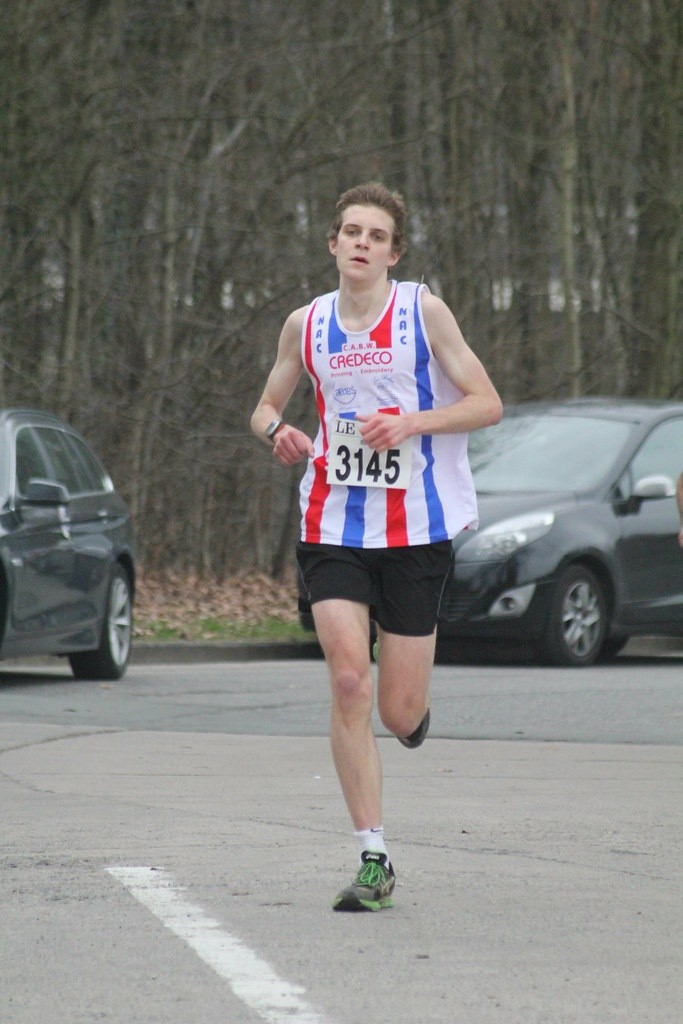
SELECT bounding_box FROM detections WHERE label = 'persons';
[249,181,505,914]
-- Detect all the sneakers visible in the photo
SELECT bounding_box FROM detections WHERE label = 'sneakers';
[333,861,395,912]
[396,710,430,748]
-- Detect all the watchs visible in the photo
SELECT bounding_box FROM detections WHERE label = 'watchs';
[265,420,285,442]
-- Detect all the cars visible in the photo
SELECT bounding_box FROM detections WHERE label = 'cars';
[295,394,683,669]
[0,406,141,682]
